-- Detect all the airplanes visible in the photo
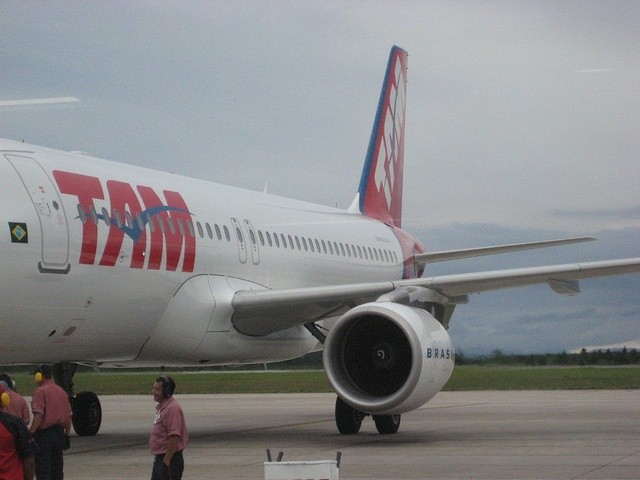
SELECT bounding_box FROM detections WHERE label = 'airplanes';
[0,43,640,435]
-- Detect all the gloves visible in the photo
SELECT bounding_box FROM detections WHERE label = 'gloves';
[63,433,69,450]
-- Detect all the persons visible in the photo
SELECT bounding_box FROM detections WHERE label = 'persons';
[27,363,73,480]
[1,385,36,480]
[1,374,29,431]
[148,376,188,480]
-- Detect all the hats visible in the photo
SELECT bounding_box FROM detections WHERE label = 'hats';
[29,363,53,375]
